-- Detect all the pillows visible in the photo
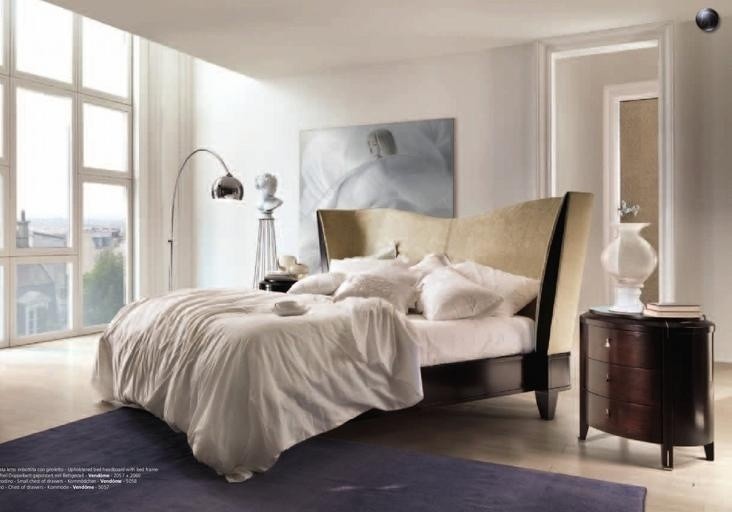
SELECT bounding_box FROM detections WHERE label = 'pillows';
[284,239,544,318]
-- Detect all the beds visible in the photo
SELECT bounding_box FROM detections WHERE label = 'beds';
[90,187,598,486]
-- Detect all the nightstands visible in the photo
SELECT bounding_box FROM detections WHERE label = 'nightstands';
[259,276,299,293]
[576,305,720,469]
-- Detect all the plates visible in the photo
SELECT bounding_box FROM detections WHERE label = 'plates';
[272,306,308,316]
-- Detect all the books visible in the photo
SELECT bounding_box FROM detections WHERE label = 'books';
[646,303,701,312]
[642,310,702,318]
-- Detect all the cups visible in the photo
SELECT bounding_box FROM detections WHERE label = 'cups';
[278,254,297,272]
[276,301,298,311]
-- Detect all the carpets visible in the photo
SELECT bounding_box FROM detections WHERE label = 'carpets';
[0,403,646,512]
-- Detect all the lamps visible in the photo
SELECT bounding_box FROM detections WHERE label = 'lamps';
[166,145,245,292]
